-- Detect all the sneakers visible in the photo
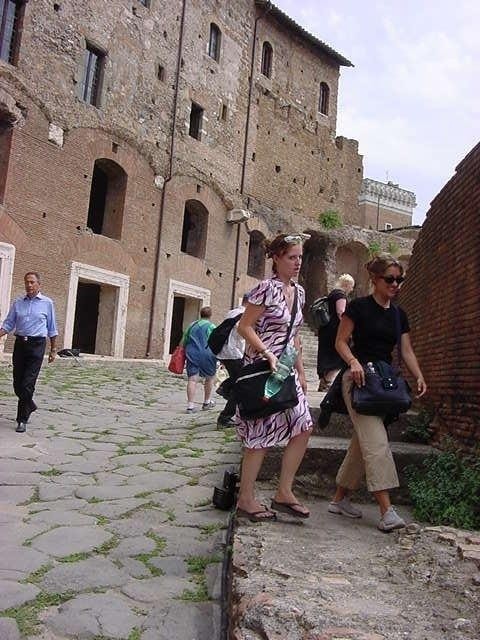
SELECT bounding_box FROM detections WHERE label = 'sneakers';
[377,507,406,532]
[202,400,216,410]
[187,407,198,414]
[328,496,362,518]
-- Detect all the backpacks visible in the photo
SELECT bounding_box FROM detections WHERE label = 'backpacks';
[308,291,345,329]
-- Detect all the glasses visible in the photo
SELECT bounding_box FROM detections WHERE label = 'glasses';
[278,235,303,248]
[379,275,405,284]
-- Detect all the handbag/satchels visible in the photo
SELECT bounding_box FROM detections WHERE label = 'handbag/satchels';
[205,313,242,355]
[351,361,413,415]
[168,346,185,374]
[233,355,299,417]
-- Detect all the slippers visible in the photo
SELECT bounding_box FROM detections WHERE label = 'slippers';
[236,505,277,522]
[271,500,310,518]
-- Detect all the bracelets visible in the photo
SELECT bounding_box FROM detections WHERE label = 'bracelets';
[50,349,57,354]
[262,348,274,357]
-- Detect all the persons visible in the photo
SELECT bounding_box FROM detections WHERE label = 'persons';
[212,291,250,430]
[0,271,58,433]
[236,234,312,523]
[180,306,226,415]
[315,271,357,392]
[326,250,428,533]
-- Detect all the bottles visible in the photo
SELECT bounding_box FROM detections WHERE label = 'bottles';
[263,344,300,403]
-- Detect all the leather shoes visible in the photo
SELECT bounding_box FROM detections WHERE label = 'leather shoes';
[16,422,25,431]
[218,415,235,429]
[216,386,230,400]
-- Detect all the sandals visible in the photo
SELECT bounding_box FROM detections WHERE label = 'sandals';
[318,376,332,392]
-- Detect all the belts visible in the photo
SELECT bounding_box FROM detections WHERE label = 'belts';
[16,336,45,341]
